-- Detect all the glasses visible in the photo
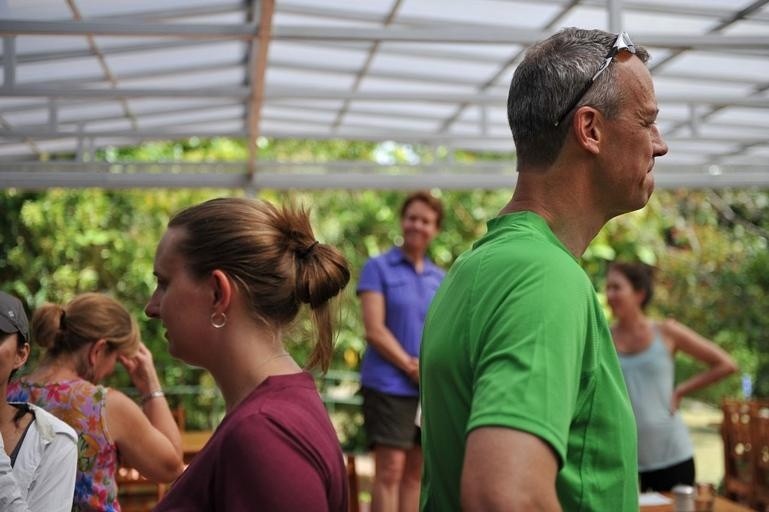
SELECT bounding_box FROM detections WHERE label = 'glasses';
[554,33,636,127]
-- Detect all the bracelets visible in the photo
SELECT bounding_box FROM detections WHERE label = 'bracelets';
[140,391,165,402]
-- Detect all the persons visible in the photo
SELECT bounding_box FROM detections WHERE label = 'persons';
[144,198,350,511]
[356,190,446,512]
[0,289,78,512]
[7,292,183,512]
[419,27,668,512]
[606,261,740,492]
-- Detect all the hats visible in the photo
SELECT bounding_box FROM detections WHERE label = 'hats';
[0,290,29,342]
